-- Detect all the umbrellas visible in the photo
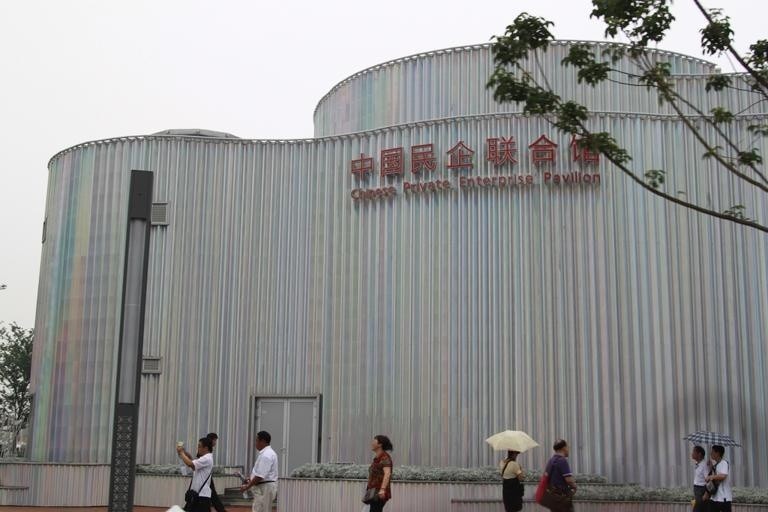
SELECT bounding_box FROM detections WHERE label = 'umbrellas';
[683,431,741,447]
[485,430,539,453]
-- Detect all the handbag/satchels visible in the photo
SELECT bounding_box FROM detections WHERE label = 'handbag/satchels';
[536,473,548,502]
[185,490,199,504]
[363,488,379,504]
[520,483,524,496]
[540,487,572,512]
[705,472,719,494]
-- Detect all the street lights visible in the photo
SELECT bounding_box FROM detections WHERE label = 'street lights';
[106,169,155,512]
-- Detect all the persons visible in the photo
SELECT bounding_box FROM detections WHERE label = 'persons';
[241,431,278,512]
[196,433,227,512]
[367,435,393,512]
[545,439,577,512]
[499,450,524,512]
[705,445,732,512]
[176,438,213,512]
[691,446,709,512]
